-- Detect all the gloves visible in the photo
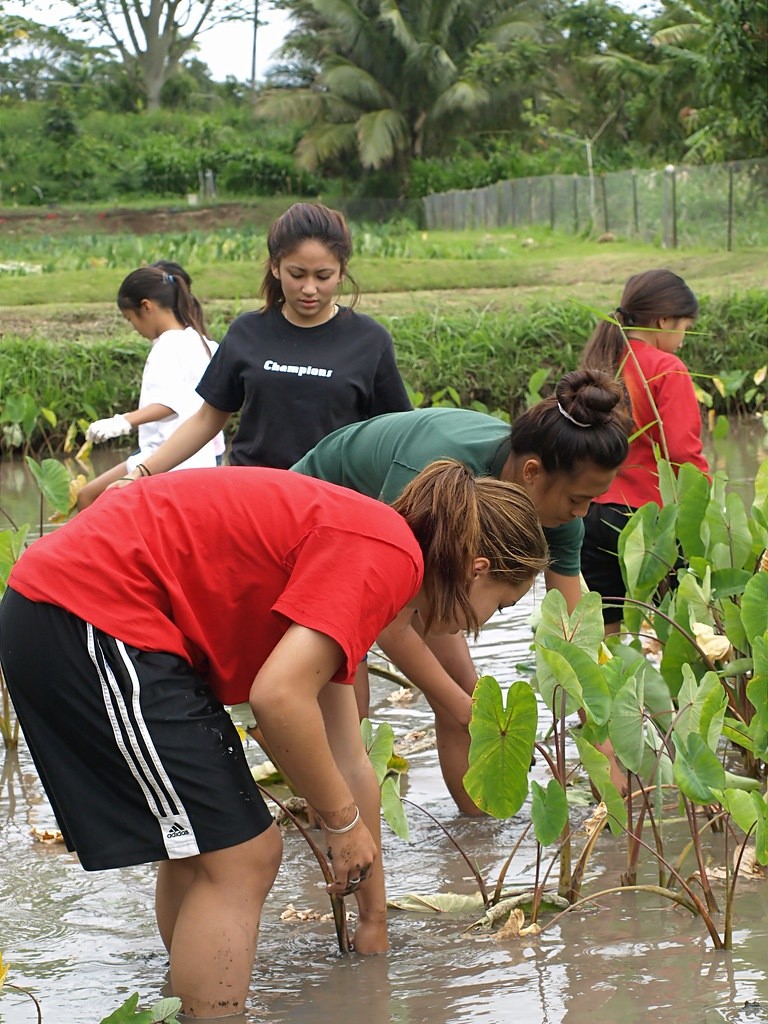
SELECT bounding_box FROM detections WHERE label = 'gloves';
[86,414,132,444]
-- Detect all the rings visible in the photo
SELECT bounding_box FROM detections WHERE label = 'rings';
[349,878,360,883]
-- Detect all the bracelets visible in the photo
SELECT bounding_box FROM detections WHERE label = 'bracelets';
[136,464,151,477]
[323,807,359,833]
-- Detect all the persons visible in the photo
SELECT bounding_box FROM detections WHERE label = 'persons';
[287,368,659,951]
[77,258,226,516]
[580,269,714,640]
[0,457,556,1021]
[106,202,415,774]
